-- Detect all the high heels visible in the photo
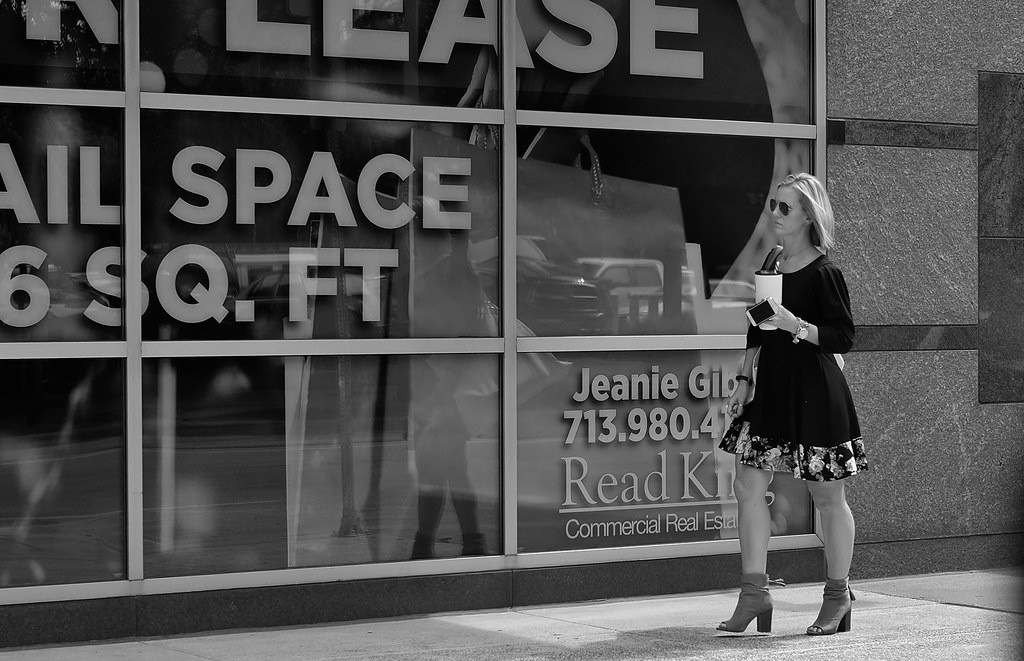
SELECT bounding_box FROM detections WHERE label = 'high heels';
[716,572,773,632]
[806,576,856,635]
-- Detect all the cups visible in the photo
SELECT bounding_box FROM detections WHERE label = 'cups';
[754,270,783,330]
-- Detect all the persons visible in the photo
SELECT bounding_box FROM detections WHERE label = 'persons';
[717,173,869,637]
[407,197,499,563]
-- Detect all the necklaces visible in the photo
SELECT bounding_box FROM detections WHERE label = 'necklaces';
[782,243,813,260]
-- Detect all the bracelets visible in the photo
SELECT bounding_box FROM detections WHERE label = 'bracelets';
[736,375,753,386]
[791,317,802,344]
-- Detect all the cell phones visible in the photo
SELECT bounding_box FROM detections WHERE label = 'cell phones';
[746,298,780,326]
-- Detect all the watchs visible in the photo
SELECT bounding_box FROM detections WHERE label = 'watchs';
[798,321,809,339]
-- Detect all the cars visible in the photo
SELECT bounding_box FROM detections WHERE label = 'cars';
[577,257,665,297]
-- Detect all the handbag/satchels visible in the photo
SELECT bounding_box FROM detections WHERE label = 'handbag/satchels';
[425,300,574,442]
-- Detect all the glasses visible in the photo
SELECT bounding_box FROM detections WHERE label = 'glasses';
[769,198,792,217]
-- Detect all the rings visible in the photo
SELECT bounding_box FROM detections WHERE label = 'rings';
[730,411,735,417]
[739,403,743,407]
[725,404,729,408]
